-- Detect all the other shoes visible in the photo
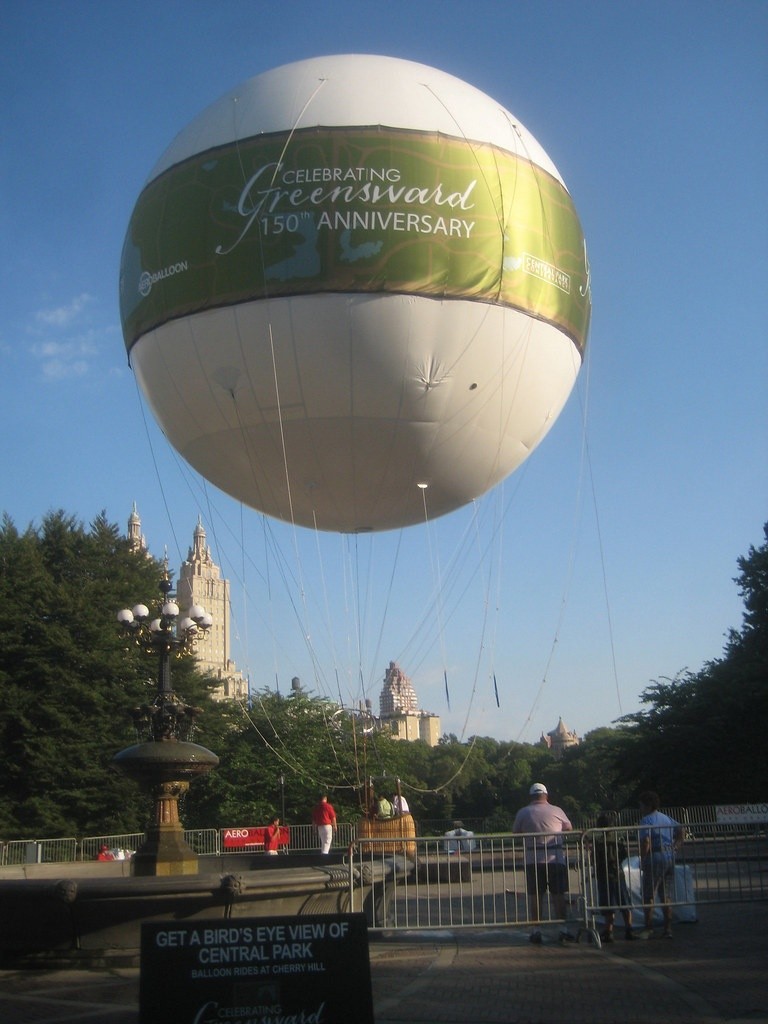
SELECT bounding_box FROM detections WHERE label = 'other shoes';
[635,929,655,939]
[601,931,614,942]
[625,926,635,939]
[660,929,672,939]
[529,929,542,942]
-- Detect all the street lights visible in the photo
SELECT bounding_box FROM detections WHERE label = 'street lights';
[113,544,221,874]
[278,769,286,825]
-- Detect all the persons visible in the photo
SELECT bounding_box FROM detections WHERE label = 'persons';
[264,815,280,855]
[390,793,409,816]
[590,813,641,943]
[512,783,579,943]
[98,846,113,861]
[636,792,685,940]
[311,794,337,854]
[377,793,391,818]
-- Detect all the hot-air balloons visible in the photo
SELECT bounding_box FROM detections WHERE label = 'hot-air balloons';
[116,50,593,852]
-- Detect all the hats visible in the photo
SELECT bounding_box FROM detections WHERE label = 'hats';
[101,845,107,851]
[529,782,548,795]
